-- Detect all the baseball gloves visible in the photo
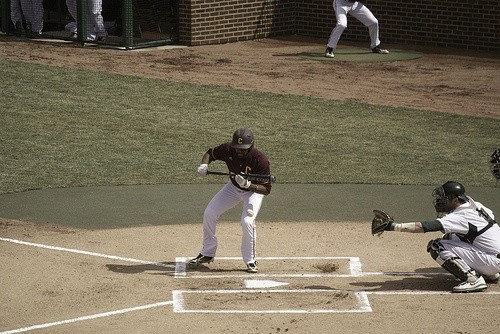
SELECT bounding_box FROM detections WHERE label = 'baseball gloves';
[372,209,394,236]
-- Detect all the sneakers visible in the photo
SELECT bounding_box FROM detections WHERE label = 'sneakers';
[484,273,499,280]
[188,253,214,266]
[453,275,488,293]
[247,261,259,272]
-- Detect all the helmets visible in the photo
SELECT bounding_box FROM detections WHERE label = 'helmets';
[435,181,465,212]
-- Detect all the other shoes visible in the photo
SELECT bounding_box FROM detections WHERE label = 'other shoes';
[86,34,108,42]
[325,47,335,58]
[372,45,391,54]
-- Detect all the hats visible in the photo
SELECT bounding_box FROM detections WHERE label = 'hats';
[231,127,255,149]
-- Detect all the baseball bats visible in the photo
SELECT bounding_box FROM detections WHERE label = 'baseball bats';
[206,171,276,183]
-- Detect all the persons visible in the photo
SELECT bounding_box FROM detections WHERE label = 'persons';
[384,181,500,292]
[9,0,110,46]
[325,0,389,58]
[490,149,500,179]
[188,128,272,273]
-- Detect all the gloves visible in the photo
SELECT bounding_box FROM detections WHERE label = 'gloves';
[197,163,209,176]
[235,172,251,189]
